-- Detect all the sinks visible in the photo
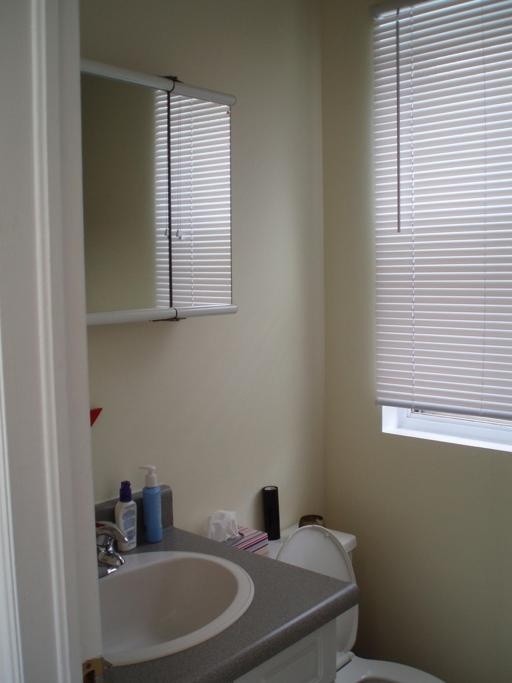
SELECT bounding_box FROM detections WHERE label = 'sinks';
[96,551,255,666]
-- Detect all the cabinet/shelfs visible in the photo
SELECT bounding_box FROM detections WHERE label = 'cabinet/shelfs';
[80,57,238,322]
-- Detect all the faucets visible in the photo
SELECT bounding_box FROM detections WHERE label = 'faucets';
[95,519,128,577]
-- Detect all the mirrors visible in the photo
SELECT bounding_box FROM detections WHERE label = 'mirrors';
[81,70,230,311]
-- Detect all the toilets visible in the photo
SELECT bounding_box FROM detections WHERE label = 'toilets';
[267,528,451,683]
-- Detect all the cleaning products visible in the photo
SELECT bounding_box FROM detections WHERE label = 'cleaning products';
[115,480,139,551]
